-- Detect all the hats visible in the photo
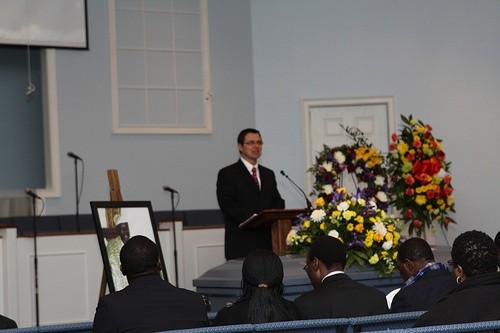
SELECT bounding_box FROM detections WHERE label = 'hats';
[113,215,128,227]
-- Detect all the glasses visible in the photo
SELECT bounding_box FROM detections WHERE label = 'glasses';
[303,255,320,272]
[243,140,263,146]
[448,260,454,267]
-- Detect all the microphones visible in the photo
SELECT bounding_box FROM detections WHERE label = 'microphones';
[163,184,179,194]
[280,170,313,207]
[67,151,82,161]
[25,188,41,200]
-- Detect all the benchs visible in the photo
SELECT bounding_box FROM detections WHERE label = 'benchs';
[0,310,500,333]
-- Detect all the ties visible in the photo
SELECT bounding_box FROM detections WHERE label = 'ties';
[251,168,259,190]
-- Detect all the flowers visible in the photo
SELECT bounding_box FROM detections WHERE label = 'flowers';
[285,113,456,279]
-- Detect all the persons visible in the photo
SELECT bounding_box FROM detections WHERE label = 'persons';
[411,230,500,328]
[92,235,211,333]
[210,249,302,327]
[291,235,392,319]
[216,128,285,262]
[389,237,461,314]
[493,230,500,271]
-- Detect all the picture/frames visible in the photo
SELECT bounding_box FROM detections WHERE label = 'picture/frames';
[90,200,169,294]
